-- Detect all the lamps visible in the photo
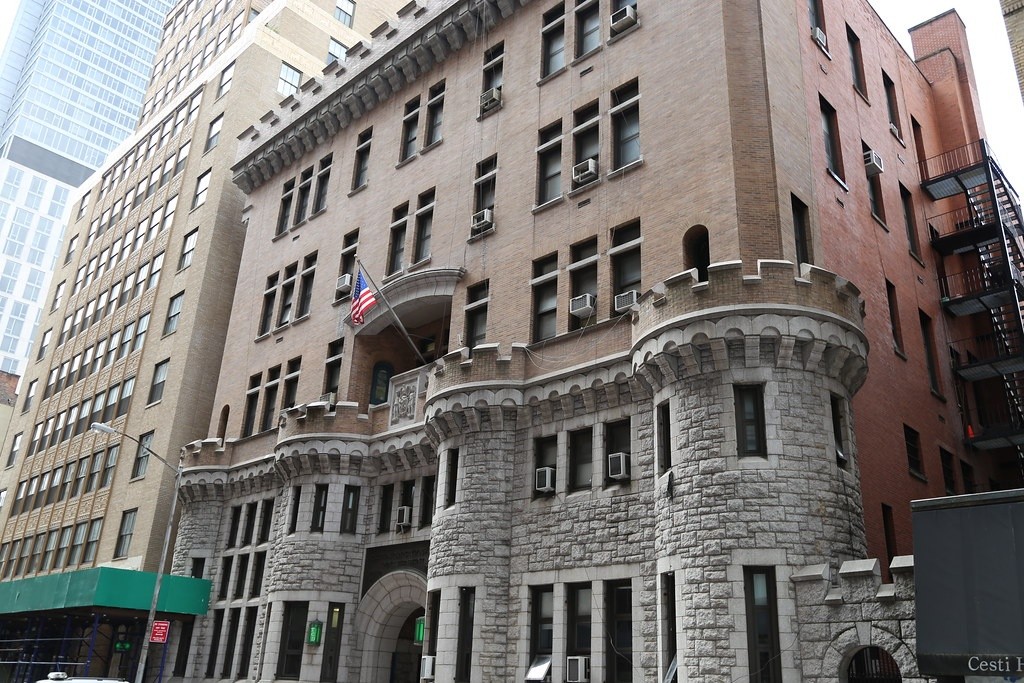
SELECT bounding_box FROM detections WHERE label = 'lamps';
[307,617,323,646]
[413,616,425,646]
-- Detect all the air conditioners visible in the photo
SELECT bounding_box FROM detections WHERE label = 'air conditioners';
[610,5,636,32]
[320,391,336,406]
[608,452,631,479]
[479,87,500,111]
[614,290,641,314]
[567,656,591,683]
[535,467,556,492]
[279,407,292,419]
[336,273,352,293]
[572,158,598,185]
[812,27,826,47]
[396,506,412,526]
[421,655,436,680]
[863,150,884,177]
[890,122,899,137]
[471,208,493,232]
[570,293,597,319]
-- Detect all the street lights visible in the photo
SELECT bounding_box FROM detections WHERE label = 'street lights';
[90,421,184,683]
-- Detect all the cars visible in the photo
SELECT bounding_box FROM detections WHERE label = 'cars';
[36,671,129,683]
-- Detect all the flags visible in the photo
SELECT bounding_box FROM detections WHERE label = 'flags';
[350,269,377,326]
